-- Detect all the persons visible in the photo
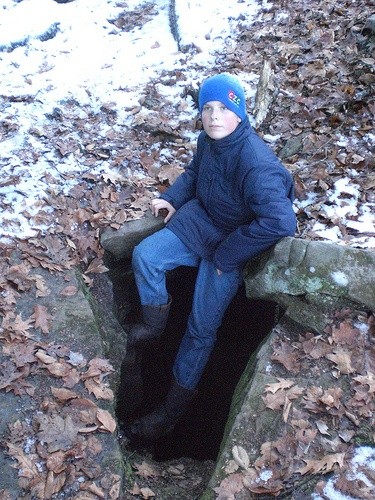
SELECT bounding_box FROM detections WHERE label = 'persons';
[122,74,295,443]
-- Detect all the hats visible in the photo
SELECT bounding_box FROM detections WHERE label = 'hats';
[198,74,245,122]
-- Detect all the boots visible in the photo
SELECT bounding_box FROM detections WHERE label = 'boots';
[138,377,197,437]
[118,293,172,345]
